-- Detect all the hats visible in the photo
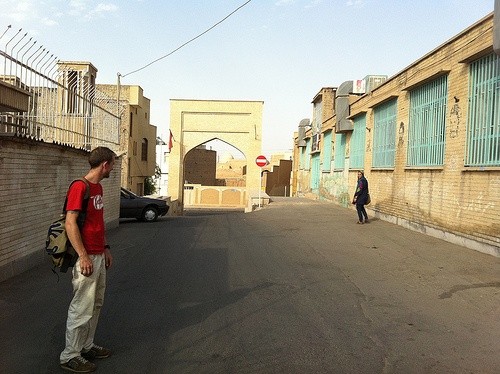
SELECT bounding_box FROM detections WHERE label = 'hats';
[359,170,364,174]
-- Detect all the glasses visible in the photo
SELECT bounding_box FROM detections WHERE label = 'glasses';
[358,174,362,175]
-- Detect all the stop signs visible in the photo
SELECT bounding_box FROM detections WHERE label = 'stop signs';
[256,155,267,167]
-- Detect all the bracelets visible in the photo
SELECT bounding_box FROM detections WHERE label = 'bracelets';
[105,245,110,250]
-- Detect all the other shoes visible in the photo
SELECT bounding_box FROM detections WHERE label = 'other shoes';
[365,219,369,223]
[356,220,362,224]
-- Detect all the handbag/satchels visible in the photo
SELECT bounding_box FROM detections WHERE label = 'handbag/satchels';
[365,194,371,205]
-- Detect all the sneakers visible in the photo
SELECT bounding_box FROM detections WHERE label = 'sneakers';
[81,342,112,358]
[61,356,96,372]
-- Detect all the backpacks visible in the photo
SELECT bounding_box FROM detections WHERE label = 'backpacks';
[45,178,91,274]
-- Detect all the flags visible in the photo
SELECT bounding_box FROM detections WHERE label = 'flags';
[169,131,173,152]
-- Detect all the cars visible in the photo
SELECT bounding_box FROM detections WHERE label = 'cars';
[120,187,170,222]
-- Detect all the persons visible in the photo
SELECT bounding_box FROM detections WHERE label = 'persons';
[352,170,369,224]
[59,146,115,372]
